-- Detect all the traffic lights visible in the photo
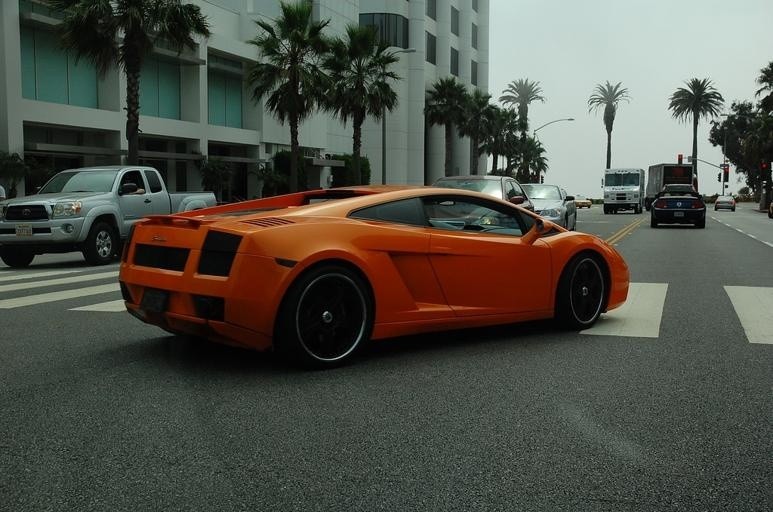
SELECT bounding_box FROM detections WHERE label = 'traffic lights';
[678,155,682,164]
[717,173,721,182]
[541,176,544,183]
[724,168,728,181]
[761,163,766,177]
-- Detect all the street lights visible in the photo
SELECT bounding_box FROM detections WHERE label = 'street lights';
[722,125,728,196]
[531,118,575,143]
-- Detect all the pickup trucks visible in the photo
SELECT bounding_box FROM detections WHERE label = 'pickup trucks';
[0,166,218,267]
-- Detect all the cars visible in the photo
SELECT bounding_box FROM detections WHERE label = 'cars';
[597,162,737,227]
[768,200,773,219]
[0,186,5,202]
[420,174,590,243]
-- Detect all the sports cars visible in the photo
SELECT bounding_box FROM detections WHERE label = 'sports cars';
[124,187,629,366]
